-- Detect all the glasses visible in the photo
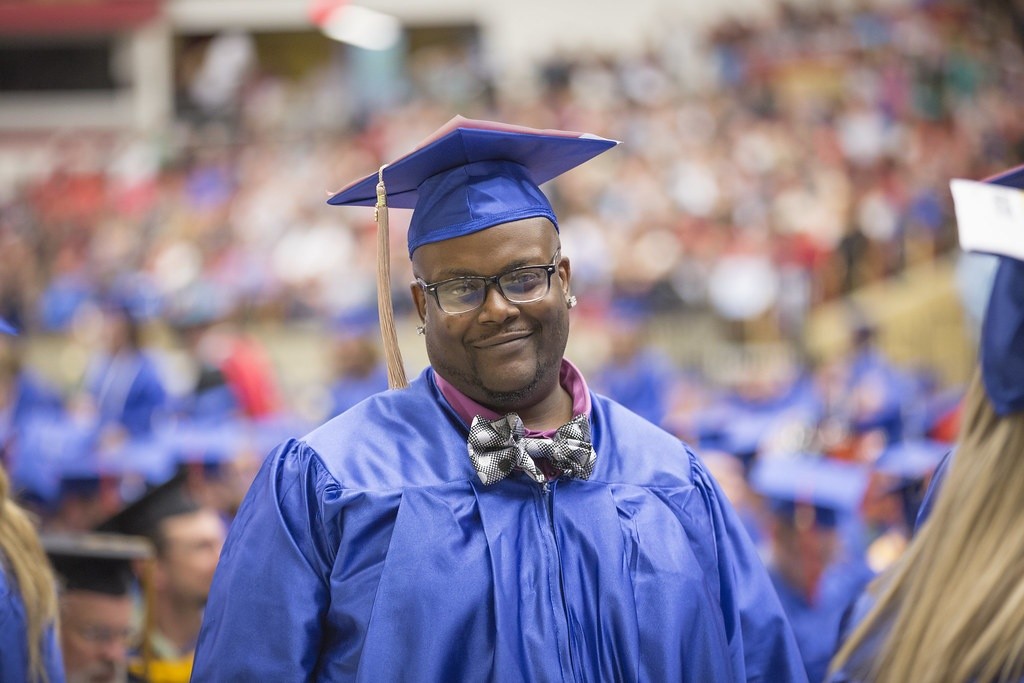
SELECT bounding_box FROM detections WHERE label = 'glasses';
[417,247,561,315]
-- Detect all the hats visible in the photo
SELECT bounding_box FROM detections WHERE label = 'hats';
[951,165,1024,260]
[327,116,620,389]
[94,469,198,530]
[605,342,960,531]
[37,536,150,593]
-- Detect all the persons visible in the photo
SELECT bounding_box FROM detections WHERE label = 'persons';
[190,158,809,682]
[0,0,1024,683]
